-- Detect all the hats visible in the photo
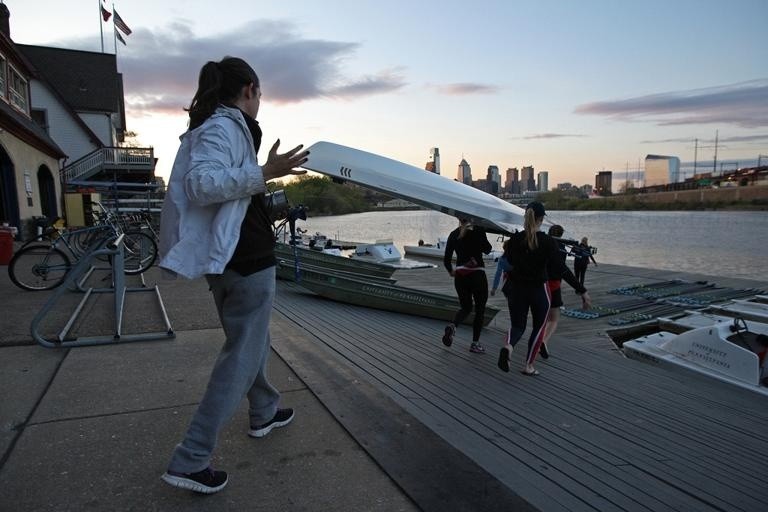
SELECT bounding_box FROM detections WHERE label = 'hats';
[525,200,549,217]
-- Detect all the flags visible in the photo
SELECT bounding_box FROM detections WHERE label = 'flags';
[115,29,126,46]
[113,9,131,36]
[101,4,111,21]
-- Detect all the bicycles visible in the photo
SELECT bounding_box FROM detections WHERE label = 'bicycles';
[7,200,157,292]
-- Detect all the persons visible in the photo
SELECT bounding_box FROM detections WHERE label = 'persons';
[443,210,495,354]
[156,54,313,495]
[497,201,591,375]
[534,225,567,359]
[569,236,598,292]
[491,240,509,297]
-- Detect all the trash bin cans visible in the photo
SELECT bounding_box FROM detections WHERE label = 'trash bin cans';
[0,227,17,265]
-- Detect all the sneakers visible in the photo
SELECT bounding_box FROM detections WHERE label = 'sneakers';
[248,405,295,439]
[159,461,231,494]
[441,323,457,348]
[469,342,487,355]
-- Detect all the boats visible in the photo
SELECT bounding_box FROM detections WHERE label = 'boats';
[276,228,441,270]
[292,141,580,248]
[623,292,768,397]
[405,238,504,261]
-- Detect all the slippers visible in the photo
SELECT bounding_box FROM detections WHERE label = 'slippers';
[496,346,512,374]
[537,341,550,361]
[520,367,542,377]
